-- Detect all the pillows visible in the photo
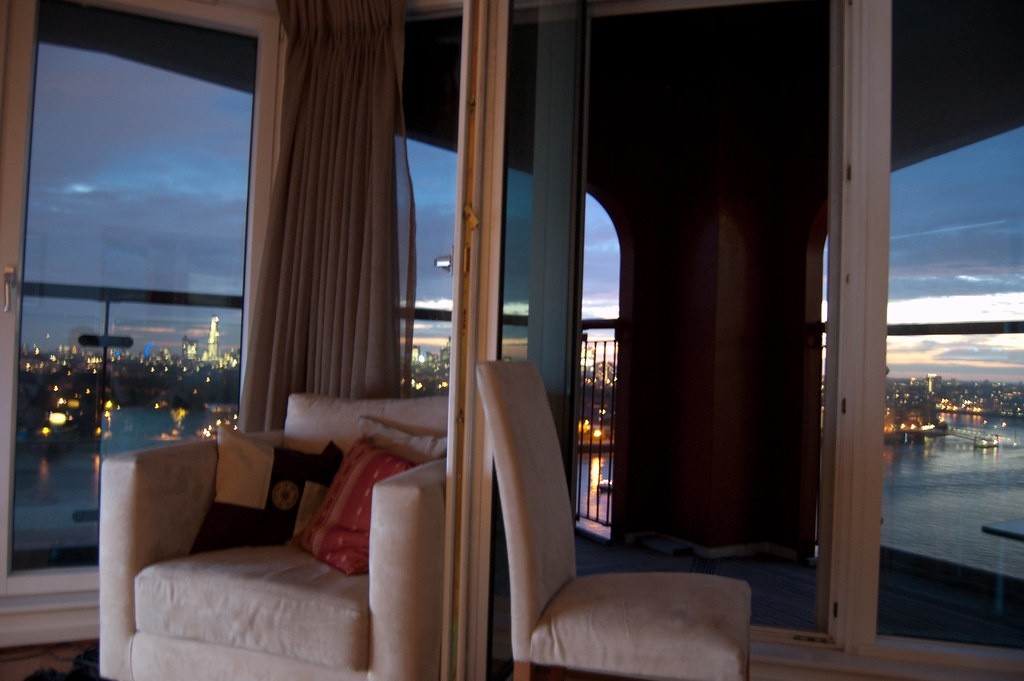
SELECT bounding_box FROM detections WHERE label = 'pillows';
[189,423,343,556]
[355,417,447,466]
[286,432,421,575]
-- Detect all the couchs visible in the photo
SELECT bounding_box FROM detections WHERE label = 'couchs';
[99,392,448,681]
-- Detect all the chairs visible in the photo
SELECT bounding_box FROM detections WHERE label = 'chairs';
[474,361,752,680]
